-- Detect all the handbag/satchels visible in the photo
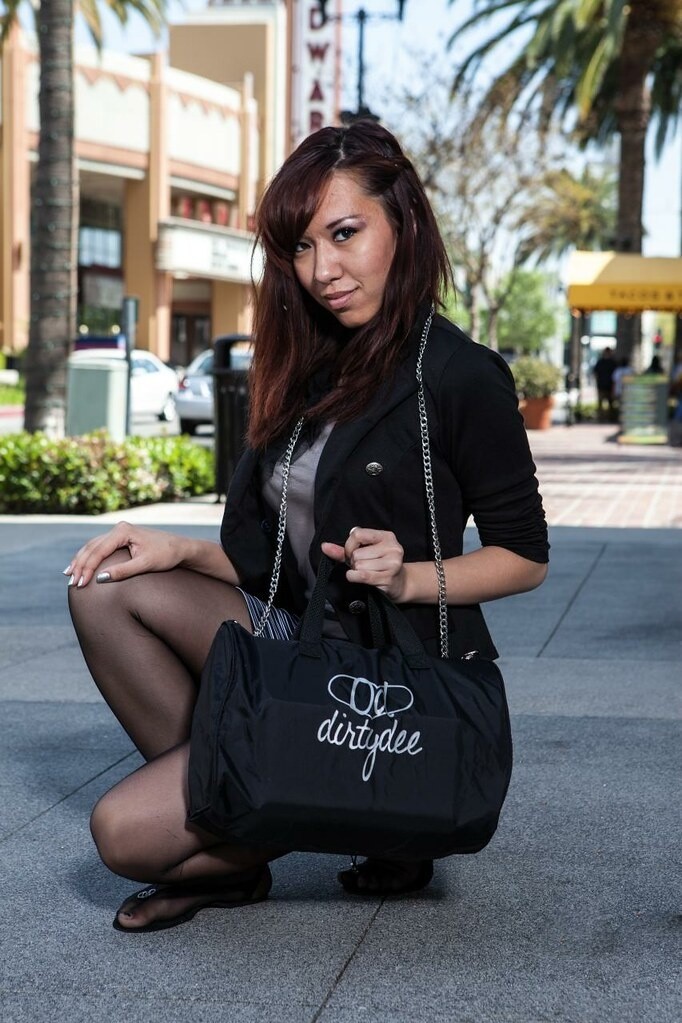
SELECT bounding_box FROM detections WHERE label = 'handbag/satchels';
[186,554,513,859]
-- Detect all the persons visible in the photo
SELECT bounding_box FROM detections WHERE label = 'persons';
[612,356,636,399]
[592,348,617,420]
[60,123,554,935]
[642,355,663,374]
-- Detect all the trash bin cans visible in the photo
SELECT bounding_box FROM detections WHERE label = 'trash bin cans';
[210,333,264,504]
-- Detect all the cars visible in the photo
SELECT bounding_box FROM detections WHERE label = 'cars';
[174,347,256,436]
[73,347,179,424]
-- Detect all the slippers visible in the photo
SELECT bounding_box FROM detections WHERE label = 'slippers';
[112,861,434,933]
